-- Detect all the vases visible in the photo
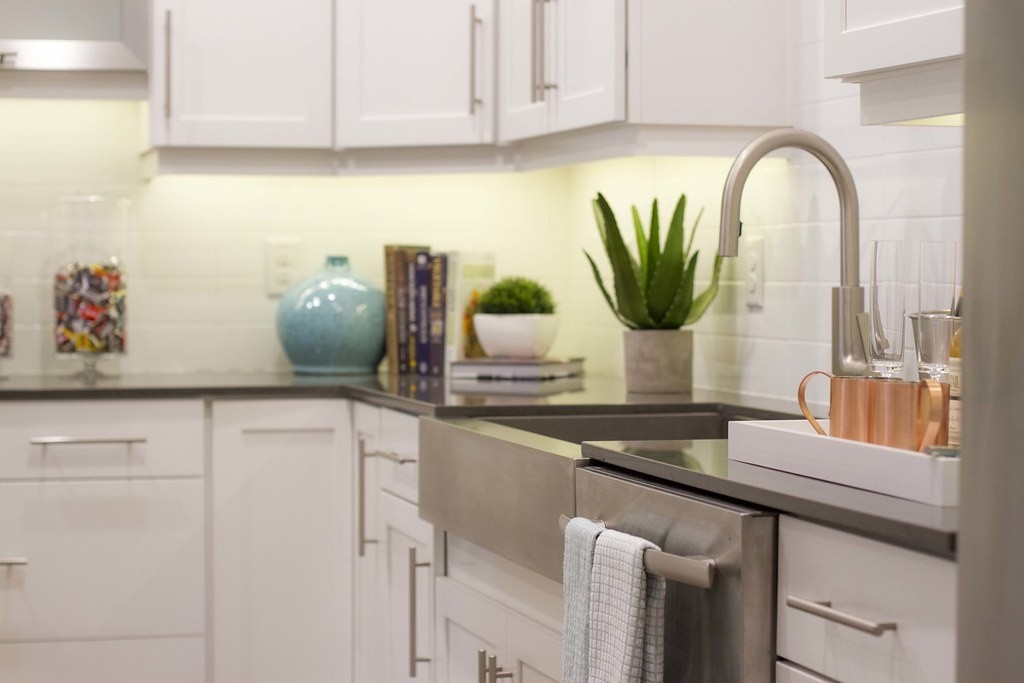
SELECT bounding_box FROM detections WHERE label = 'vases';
[274,254,391,375]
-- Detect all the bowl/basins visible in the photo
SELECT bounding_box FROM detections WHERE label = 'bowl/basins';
[909,310,963,359]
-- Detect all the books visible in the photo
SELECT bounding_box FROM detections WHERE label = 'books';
[386,245,493,376]
[451,355,586,378]
[389,375,454,406]
[452,378,582,395]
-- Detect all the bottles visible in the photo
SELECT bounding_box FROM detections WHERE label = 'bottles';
[956,281,964,317]
[276,256,386,375]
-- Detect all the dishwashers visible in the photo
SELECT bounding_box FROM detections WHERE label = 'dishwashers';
[561,463,776,683]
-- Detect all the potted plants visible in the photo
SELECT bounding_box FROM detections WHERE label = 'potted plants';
[581,192,723,396]
[473,279,556,360]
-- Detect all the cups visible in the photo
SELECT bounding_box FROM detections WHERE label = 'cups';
[798,371,903,447]
[919,241,956,373]
[869,240,906,372]
[869,379,950,456]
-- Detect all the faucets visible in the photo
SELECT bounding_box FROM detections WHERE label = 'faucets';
[717,129,875,377]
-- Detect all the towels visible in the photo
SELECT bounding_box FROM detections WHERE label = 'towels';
[562,517,665,683]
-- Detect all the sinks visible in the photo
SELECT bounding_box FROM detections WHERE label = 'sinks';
[418,410,762,587]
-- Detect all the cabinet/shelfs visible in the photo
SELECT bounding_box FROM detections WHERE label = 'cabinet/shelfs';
[145,0,849,156]
[772,512,961,683]
[0,399,580,683]
